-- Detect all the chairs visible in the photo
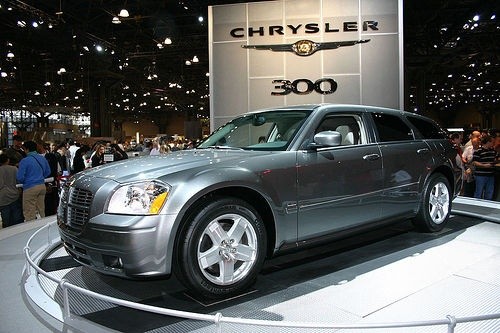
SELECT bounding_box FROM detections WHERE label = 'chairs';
[286,125,354,145]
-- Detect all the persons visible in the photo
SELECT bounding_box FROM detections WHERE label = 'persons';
[0,135,87,228]
[91,142,128,167]
[451,128,500,202]
[129,138,193,156]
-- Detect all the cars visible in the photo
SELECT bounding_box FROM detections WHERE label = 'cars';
[57,102,465,304]
